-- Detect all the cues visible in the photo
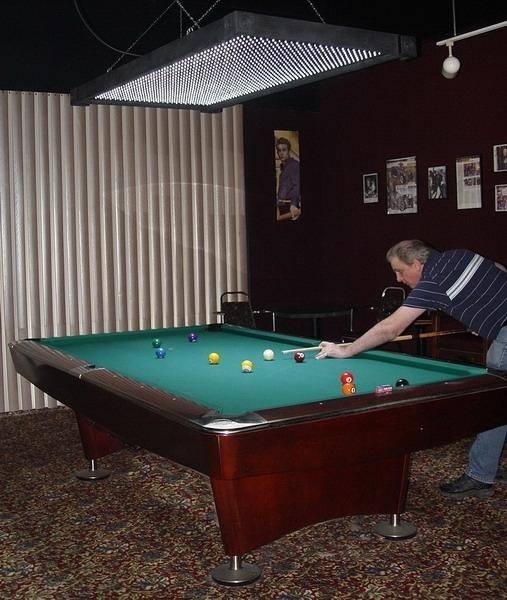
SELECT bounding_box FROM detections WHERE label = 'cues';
[282,327,480,355]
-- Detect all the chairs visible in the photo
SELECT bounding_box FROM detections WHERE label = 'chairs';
[343,287,405,352]
[221,292,275,332]
[252,304,298,336]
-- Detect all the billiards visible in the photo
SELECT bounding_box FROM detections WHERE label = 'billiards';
[156,349,165,357]
[294,352,304,362]
[188,333,197,342]
[342,384,356,396]
[397,378,409,388]
[152,338,161,348]
[341,372,353,383]
[263,350,274,360]
[208,353,219,365]
[242,360,253,372]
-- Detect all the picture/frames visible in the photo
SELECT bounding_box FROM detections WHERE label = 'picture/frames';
[455,156,483,211]
[494,184,507,213]
[362,172,379,204]
[385,154,420,215]
[493,143,507,173]
[272,125,304,225]
[427,164,447,200]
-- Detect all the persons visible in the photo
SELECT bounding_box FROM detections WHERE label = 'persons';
[317,239,507,497]
[275,137,300,220]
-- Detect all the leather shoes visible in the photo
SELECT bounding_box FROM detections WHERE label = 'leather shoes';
[437,475,495,500]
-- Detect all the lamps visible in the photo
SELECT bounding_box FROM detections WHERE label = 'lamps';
[442,42,461,73]
[69,11,418,114]
[441,69,456,78]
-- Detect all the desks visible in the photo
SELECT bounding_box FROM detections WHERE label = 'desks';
[272,310,350,339]
